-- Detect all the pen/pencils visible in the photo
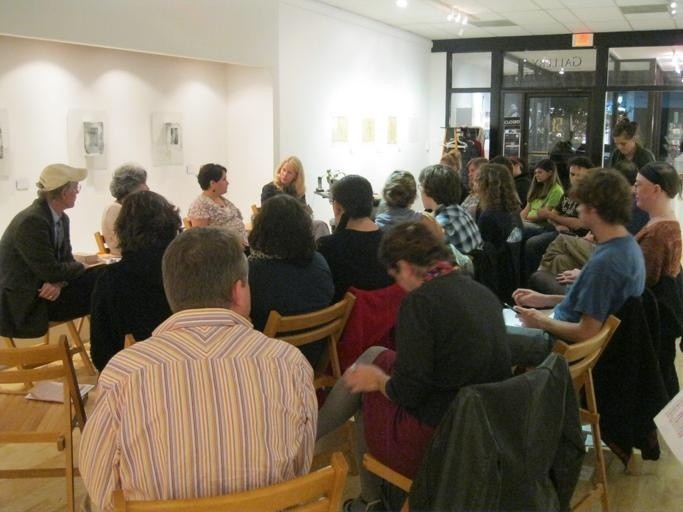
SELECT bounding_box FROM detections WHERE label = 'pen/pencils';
[504,303,521,314]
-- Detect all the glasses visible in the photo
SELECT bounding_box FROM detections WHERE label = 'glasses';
[65,182,82,192]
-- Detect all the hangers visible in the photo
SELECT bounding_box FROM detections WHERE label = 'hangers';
[442,133,468,154]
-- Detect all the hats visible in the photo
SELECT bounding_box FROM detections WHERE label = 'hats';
[36,163,88,193]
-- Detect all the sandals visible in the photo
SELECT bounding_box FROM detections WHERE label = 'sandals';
[341,493,384,512]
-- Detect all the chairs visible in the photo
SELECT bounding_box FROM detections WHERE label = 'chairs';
[1,204,681,510]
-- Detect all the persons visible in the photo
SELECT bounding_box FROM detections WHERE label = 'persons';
[606,118,655,169]
[0,162,125,372]
